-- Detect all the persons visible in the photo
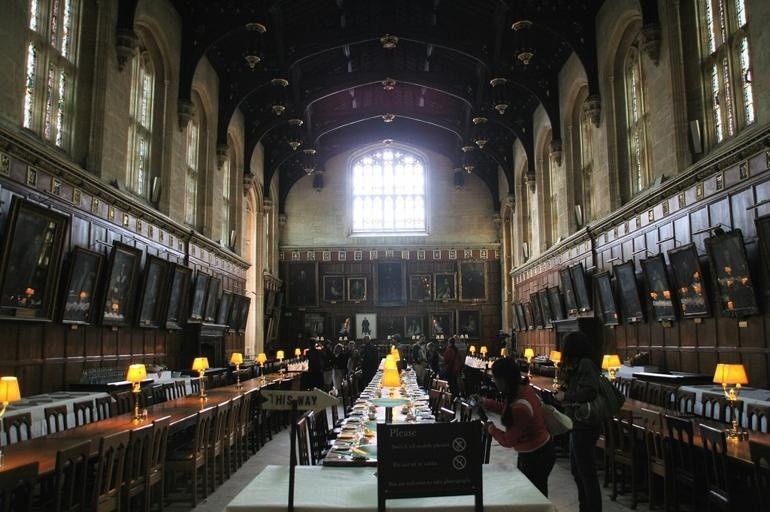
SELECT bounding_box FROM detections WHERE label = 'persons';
[468,358,557,499]
[551,339,606,512]
[304,331,461,398]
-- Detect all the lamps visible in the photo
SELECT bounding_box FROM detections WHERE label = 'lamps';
[454,18,537,191]
[243,23,326,193]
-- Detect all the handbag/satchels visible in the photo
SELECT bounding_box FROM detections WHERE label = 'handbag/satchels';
[573,374,625,433]
[535,392,573,436]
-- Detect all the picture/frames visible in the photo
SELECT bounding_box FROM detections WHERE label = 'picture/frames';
[284,258,488,341]
[510,198,769,334]
[2,195,252,335]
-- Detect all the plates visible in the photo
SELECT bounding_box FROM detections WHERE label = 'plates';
[333,367,432,451]
